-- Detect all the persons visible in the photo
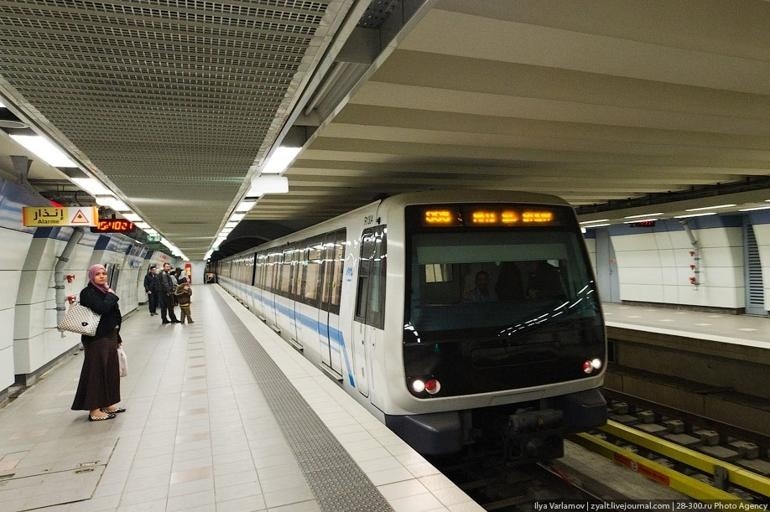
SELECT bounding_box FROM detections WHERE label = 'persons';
[144,262,195,325]
[466,271,498,303]
[69,263,128,422]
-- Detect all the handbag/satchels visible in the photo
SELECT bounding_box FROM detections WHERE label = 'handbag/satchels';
[115,349,129,378]
[58,306,100,338]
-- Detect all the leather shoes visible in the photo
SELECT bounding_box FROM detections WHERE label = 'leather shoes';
[87,406,127,422]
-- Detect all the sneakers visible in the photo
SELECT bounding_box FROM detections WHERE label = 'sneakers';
[149,312,194,326]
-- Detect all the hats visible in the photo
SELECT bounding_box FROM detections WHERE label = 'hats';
[173,267,184,274]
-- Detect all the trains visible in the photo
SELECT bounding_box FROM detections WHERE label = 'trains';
[206,190,608,467]
